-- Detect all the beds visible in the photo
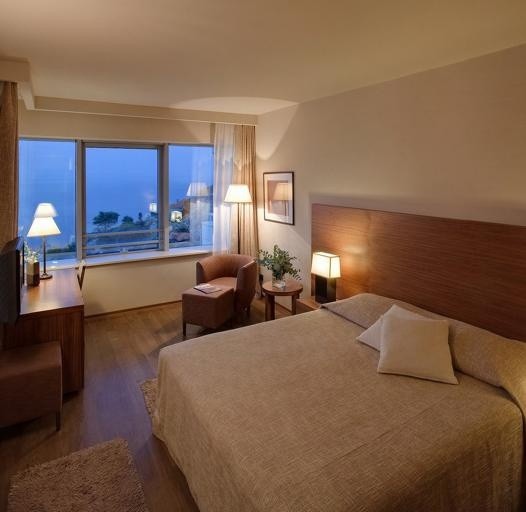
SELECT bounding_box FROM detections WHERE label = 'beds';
[143,203,525,511]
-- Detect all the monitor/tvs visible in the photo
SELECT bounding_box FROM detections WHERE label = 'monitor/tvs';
[0,237,27,322]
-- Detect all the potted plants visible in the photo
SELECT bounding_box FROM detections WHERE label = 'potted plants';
[254,246,302,290]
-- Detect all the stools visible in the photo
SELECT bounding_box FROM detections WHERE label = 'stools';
[0,341,63,432]
[181,283,235,335]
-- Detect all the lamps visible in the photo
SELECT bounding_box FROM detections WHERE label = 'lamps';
[32,202,57,219]
[220,184,254,254]
[25,217,61,280]
[181,182,209,250]
[310,252,341,304]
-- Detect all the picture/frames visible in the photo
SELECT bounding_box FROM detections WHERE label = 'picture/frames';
[262,170,295,226]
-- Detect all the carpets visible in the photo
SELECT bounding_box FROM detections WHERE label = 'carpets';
[7,440,150,512]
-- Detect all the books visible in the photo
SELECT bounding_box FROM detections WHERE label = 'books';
[194,282,223,295]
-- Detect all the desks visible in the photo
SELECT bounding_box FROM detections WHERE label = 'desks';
[261,280,302,321]
[5,268,85,388]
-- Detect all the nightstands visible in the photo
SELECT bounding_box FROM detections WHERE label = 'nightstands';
[294,297,339,315]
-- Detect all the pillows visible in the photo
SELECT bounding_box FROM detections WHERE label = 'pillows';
[355,303,435,353]
[377,315,460,386]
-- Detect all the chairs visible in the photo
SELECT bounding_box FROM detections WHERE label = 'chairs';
[195,250,255,315]
[77,260,86,285]
